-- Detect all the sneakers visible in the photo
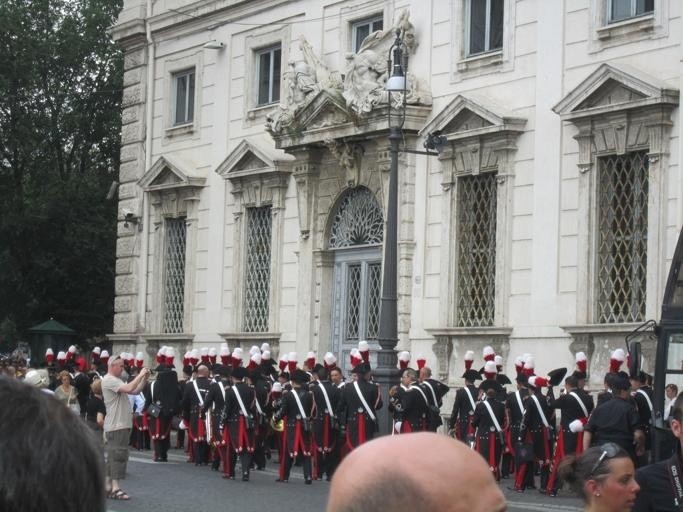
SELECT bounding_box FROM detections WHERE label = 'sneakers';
[276,475,329,485]
[195,462,267,481]
[507,484,561,498]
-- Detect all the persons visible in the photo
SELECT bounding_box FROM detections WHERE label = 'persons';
[0,370,105,510]
[631,390,682,511]
[559,443,639,511]
[388,347,682,495]
[328,430,512,512]
[44,342,384,484]
[100,352,151,502]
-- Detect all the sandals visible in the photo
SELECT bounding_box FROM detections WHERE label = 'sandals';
[107,488,130,500]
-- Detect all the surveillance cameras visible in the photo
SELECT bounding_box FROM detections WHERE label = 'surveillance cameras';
[121,209,132,218]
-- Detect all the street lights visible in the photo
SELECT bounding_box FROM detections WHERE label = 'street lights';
[367,28,410,439]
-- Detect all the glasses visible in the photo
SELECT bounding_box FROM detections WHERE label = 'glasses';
[109,355,121,364]
[588,441,621,480]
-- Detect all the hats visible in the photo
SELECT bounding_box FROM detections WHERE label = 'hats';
[120,341,374,380]
[460,349,483,381]
[603,348,648,388]
[397,350,432,377]
[45,344,110,371]
[547,367,568,386]
[572,351,587,379]
[478,345,548,390]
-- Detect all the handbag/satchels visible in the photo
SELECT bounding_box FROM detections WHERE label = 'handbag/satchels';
[65,386,82,416]
